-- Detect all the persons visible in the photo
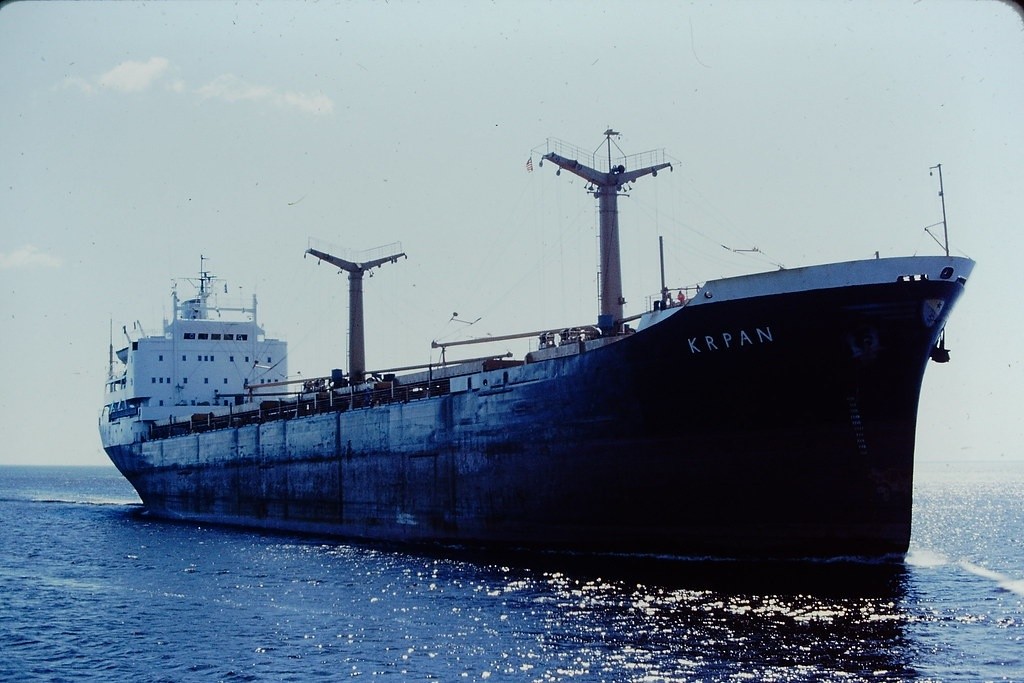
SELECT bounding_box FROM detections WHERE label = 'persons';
[676,291,685,303]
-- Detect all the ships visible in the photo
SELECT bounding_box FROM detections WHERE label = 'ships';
[97,127,978,562]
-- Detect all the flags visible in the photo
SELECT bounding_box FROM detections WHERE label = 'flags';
[526,158,533,173]
